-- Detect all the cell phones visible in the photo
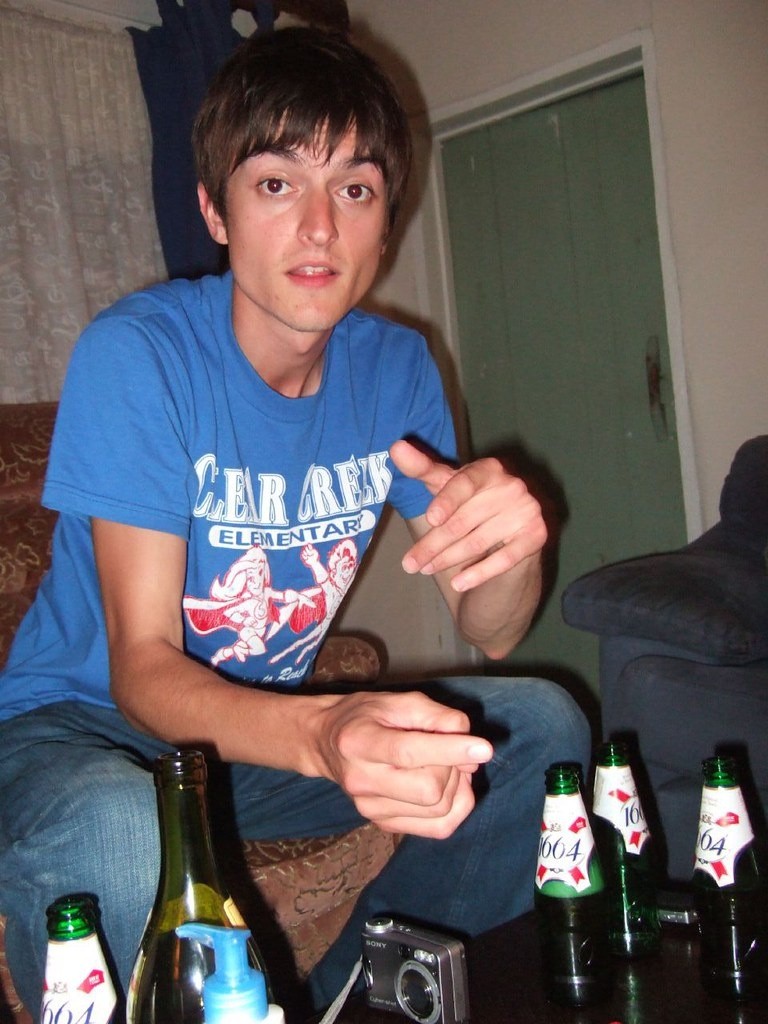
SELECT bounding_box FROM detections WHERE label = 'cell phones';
[656,890,699,925]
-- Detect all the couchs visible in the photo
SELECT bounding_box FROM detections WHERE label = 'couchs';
[1,404,406,1021]
[561,434,767,881]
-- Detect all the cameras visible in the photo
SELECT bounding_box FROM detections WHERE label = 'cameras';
[360,917,471,1024]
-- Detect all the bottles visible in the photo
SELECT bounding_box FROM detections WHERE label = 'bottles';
[533,764,611,1007]
[693,754,766,1002]
[126,749,270,1024]
[41,897,127,1024]
[586,743,666,957]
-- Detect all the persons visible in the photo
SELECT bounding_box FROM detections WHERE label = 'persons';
[0,27,591,1024]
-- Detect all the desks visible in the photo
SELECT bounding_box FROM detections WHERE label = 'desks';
[311,890,767,1023]
